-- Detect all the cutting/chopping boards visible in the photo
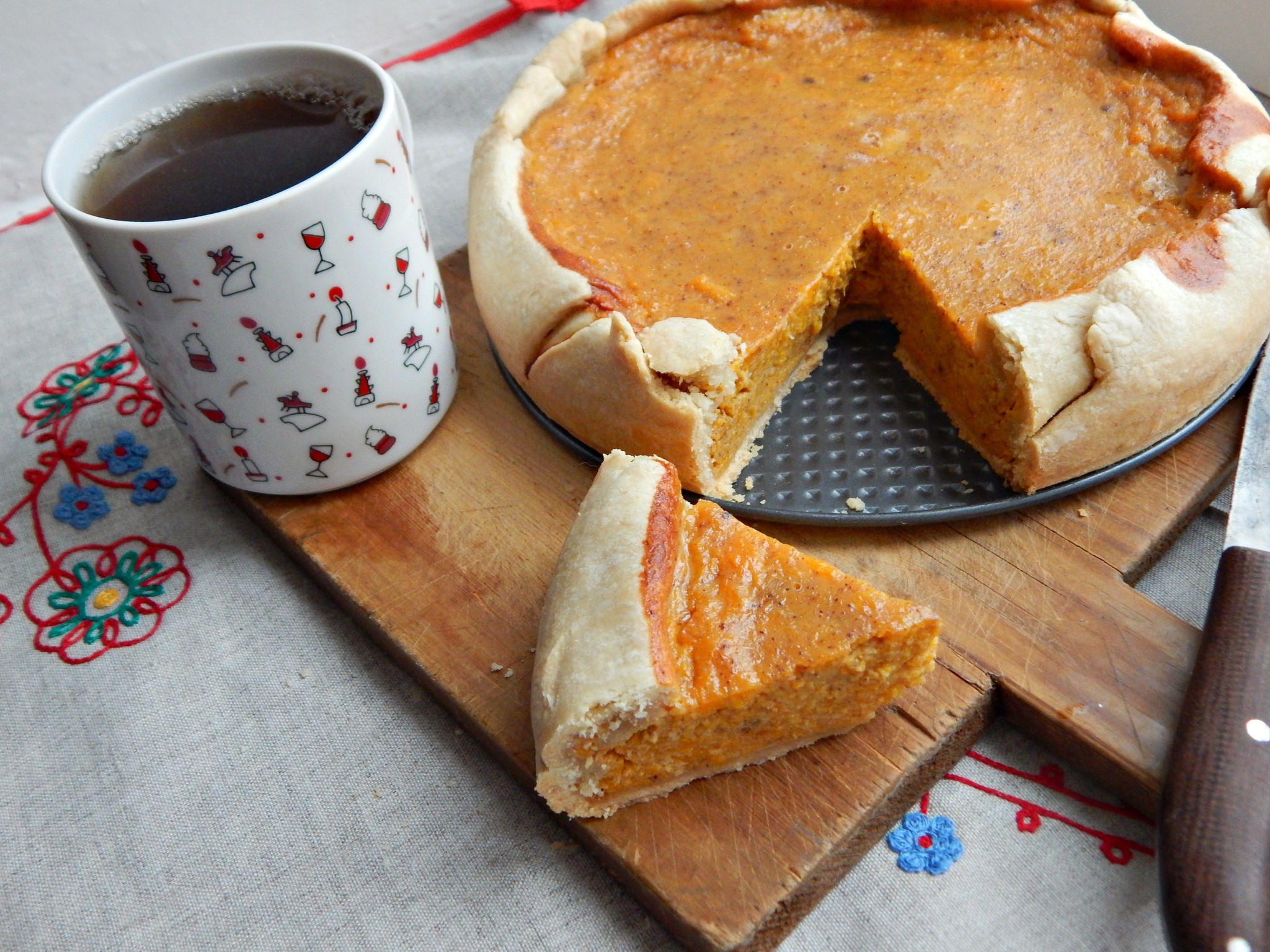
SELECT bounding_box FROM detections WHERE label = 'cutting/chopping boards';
[227,238,1254,951]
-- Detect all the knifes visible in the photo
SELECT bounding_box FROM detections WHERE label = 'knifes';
[1158,335,1270,951]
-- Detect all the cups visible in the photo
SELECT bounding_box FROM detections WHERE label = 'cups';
[40,42,457,496]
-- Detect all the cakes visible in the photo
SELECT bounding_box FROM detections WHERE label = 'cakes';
[466,0,1266,814]
